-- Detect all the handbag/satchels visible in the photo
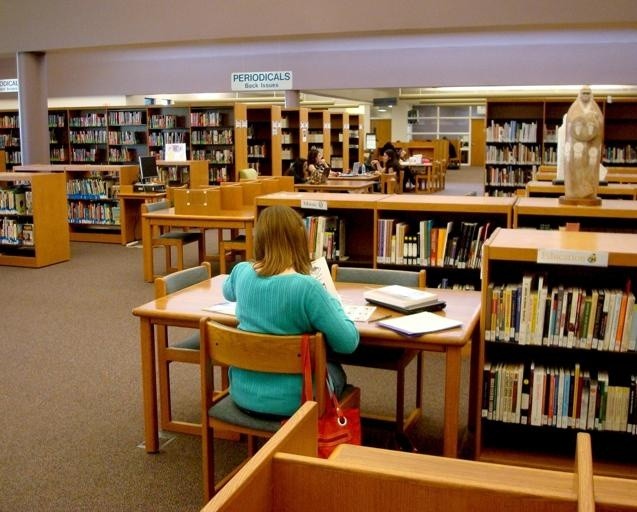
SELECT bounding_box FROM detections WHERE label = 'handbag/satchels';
[280,408,362,459]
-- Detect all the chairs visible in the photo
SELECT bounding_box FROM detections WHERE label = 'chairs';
[153,260,241,442]
[331,263,427,440]
[140,198,203,272]
[219,236,245,274]
[380,160,448,192]
[199,315,362,506]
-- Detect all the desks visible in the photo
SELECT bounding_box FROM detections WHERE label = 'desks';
[329,174,380,180]
[294,180,376,193]
[131,273,481,458]
[142,176,295,282]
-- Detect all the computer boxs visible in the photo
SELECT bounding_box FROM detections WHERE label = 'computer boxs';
[135,183,166,192]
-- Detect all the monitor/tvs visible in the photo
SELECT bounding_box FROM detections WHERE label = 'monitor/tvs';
[139,157,158,184]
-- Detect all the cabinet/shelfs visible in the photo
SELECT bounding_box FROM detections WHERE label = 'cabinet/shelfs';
[395,139,462,167]
[255,191,517,290]
[485,97,637,199]
[513,197,637,234]
[480,227,637,478]
[0,172,71,267]
[0,105,365,186]
[12,164,142,244]
[200,401,637,511]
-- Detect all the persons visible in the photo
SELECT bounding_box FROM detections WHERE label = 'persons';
[307,149,331,179]
[222,206,359,422]
[283,159,308,184]
[565,86,605,199]
[371,142,416,194]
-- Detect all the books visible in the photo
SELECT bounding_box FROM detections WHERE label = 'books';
[364,285,446,315]
[485,275,636,353]
[1,111,266,246]
[377,219,491,269]
[486,119,636,199]
[377,311,463,335]
[438,278,475,291]
[302,216,346,262]
[482,362,636,435]
